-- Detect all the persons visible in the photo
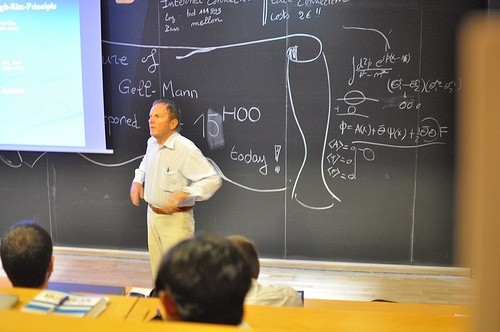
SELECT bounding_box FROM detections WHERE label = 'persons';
[226,235,304,307]
[0,220,55,289]
[155,234,252,328]
[130,98,224,280]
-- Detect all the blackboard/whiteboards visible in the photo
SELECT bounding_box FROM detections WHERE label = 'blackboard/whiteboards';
[0,1,500,277]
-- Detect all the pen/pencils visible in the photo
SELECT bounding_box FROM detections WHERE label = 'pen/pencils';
[50,296,69,312]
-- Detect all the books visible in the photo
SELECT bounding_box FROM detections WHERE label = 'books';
[20,289,110,319]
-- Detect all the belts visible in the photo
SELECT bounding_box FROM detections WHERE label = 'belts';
[150,204,193,214]
